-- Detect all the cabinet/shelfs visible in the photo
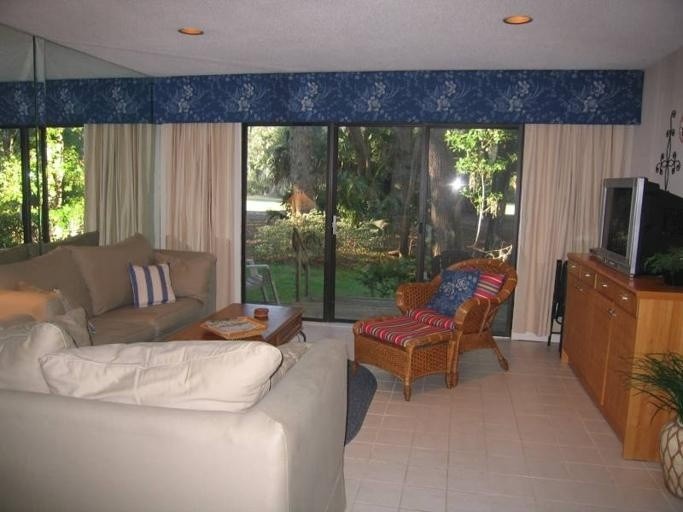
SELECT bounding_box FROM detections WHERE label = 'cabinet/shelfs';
[582,264,682,463]
[560,252,594,406]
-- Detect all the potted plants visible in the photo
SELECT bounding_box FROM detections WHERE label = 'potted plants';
[612,347,683,498]
[640,247,682,285]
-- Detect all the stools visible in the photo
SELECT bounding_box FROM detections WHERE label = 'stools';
[350,313,457,401]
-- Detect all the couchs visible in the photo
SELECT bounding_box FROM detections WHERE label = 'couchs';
[1,322,349,512]
[0,231,218,347]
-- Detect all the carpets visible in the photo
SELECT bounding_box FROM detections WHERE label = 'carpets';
[343,358,379,448]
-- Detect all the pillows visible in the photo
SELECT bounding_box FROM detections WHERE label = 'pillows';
[38,339,281,412]
[125,260,177,307]
[55,306,92,347]
[1,319,75,393]
[427,268,480,316]
[472,272,504,299]
[54,289,95,333]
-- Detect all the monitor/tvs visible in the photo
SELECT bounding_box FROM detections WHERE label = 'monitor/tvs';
[597,177,683,278]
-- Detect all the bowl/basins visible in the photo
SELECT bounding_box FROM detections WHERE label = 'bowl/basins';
[254,308,268,318]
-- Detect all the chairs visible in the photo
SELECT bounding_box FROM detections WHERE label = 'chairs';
[395,257,519,387]
[546,259,566,352]
[245,257,280,304]
